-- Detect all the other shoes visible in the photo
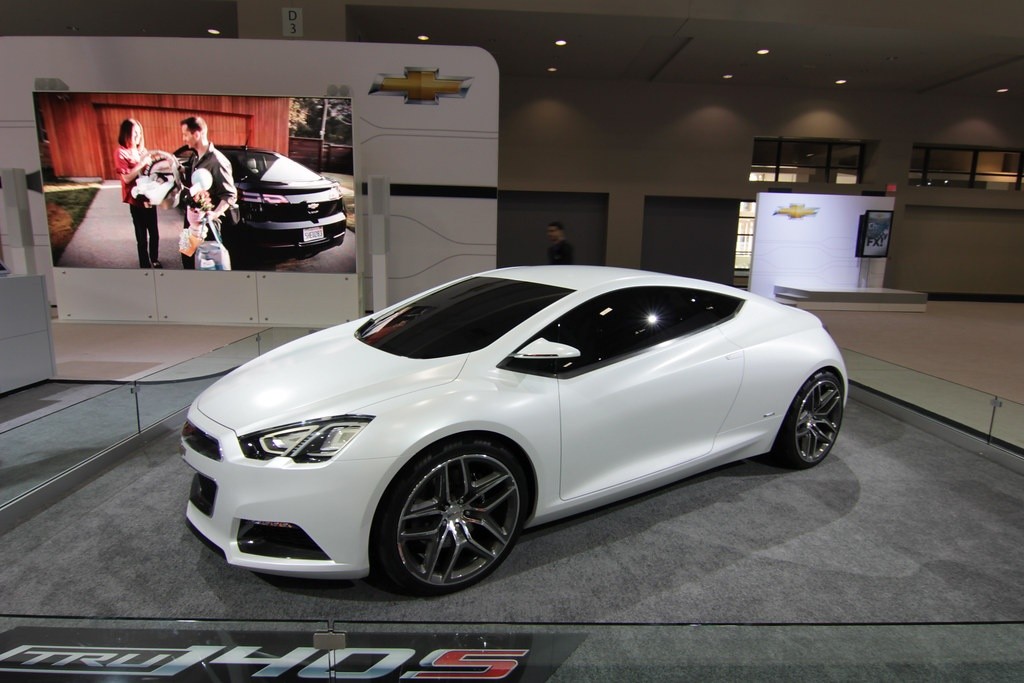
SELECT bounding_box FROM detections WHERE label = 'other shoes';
[151,260,163,268]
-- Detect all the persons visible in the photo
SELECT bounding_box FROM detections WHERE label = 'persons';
[177,116,237,269]
[115,118,163,269]
[547,222,573,265]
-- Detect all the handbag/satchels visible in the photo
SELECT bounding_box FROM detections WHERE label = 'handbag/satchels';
[195,217,231,271]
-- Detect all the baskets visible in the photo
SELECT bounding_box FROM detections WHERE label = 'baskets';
[137,151,185,210]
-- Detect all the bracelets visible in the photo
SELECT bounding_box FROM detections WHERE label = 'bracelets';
[177,165,181,170]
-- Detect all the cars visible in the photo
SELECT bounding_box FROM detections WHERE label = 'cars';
[148,144,346,267]
[178,264,848,590]
